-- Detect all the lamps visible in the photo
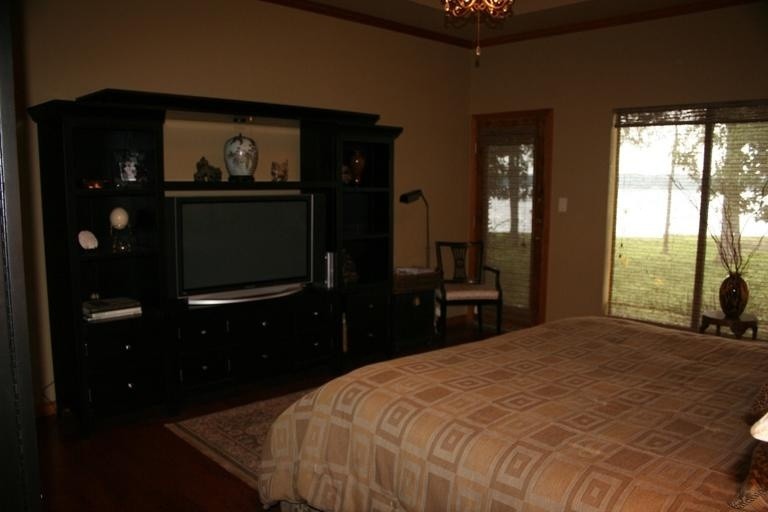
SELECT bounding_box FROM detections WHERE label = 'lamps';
[400,188,431,268]
[442,0,512,24]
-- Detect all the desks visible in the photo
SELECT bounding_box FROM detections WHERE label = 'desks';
[697,310,758,338]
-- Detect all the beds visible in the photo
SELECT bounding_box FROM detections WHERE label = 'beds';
[260,315,768,510]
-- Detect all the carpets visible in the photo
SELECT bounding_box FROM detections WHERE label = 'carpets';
[164,385,318,493]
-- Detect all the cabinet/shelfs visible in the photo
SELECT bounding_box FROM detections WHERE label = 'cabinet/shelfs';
[27,89,404,431]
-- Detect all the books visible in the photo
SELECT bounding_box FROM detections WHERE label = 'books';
[82,297,143,324]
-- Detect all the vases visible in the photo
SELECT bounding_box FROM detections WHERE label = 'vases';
[717,271,749,318]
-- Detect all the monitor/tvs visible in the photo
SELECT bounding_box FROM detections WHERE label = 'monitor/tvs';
[173,194,315,306]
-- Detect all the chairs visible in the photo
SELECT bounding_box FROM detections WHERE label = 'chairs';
[435,239,504,340]
